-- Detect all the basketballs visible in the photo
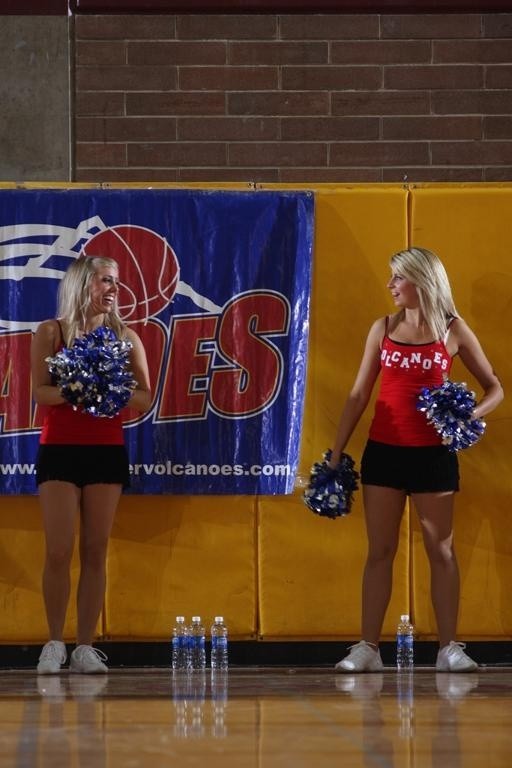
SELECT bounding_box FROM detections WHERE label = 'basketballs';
[78,224,180,325]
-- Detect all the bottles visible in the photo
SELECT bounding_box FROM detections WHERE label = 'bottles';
[210,616,229,673]
[396,614,414,674]
[171,615,190,673]
[189,615,206,673]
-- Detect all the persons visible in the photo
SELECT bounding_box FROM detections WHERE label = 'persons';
[28,252,152,674]
[323,248,508,674]
[35,674,108,768]
[335,675,481,767]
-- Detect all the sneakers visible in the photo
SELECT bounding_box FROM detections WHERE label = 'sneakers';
[335,641,383,672]
[71,645,111,672]
[38,641,67,674]
[437,641,479,672]
